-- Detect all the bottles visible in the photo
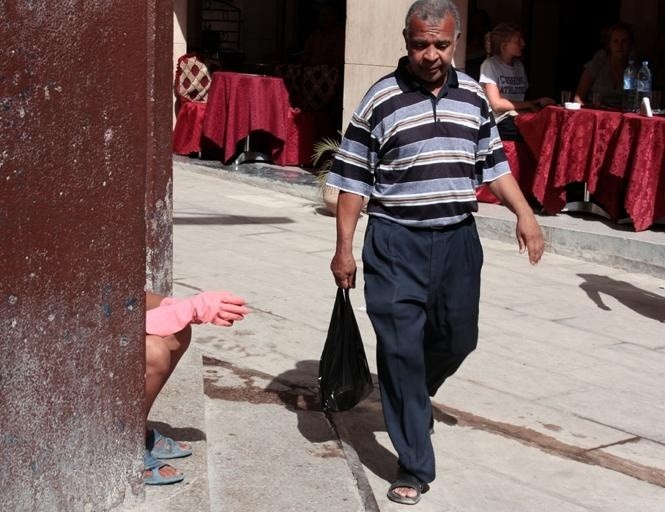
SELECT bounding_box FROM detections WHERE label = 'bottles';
[619,59,651,116]
[592,79,602,107]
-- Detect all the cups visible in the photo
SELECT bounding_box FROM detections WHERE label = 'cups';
[561,91,572,106]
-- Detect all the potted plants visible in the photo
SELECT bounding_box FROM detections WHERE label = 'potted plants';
[303,125,374,220]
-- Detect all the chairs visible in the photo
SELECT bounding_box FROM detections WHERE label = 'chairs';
[171,52,216,162]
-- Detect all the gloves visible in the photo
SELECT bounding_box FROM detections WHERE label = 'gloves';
[146,290,251,335]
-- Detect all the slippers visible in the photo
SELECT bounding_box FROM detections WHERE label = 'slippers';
[143,450,184,485]
[145,428,192,458]
[388,472,424,503]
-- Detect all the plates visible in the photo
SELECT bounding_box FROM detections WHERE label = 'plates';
[564,102,582,110]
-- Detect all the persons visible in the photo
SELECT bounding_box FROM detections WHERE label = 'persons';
[325,0,546,505]
[573,22,634,110]
[479,24,555,214]
[144,290,248,485]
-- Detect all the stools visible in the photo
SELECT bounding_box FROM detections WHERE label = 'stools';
[472,134,534,209]
